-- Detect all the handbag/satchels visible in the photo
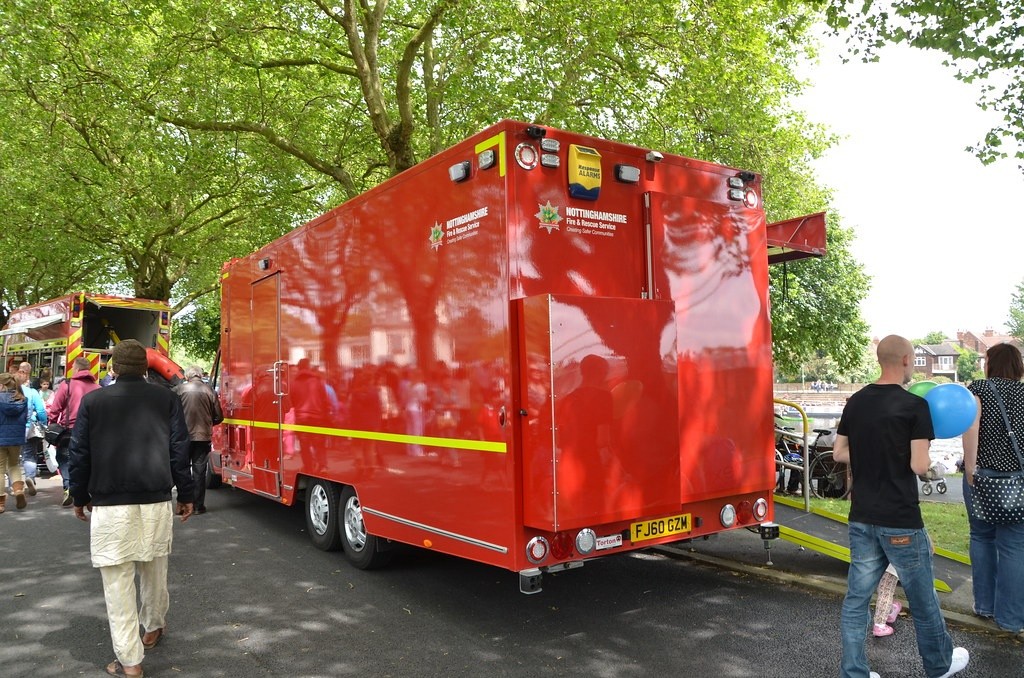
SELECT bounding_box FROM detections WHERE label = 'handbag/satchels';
[971,474,1024,524]
[26,421,45,440]
[44,423,70,448]
[42,440,59,472]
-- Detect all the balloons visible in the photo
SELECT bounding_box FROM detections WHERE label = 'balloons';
[908,381,938,397]
[924,383,978,439]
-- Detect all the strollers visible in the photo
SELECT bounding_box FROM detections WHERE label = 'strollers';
[919,461,948,495]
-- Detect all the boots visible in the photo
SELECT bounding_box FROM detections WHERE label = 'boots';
[0,495,6,513]
[12,480,26,510]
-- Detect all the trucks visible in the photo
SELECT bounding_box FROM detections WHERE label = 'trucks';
[202,117,829,596]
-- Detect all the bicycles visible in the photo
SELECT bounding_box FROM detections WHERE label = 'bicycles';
[774,427,854,501]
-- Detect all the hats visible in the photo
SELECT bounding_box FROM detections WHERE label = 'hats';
[112,339,148,375]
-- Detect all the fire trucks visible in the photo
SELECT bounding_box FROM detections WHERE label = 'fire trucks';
[0,291,184,397]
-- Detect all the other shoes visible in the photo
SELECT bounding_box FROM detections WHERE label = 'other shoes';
[25,477,37,496]
[194,505,207,515]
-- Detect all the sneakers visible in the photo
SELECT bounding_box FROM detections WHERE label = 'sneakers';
[62,488,73,506]
[870,672,880,678]
[872,622,894,636]
[936,647,969,678]
[885,601,902,623]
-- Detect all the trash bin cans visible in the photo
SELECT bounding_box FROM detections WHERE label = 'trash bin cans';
[816,446,848,501]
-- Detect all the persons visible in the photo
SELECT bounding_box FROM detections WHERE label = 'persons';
[833,335,969,678]
[100,366,115,387]
[50,358,102,506]
[222,356,506,473]
[873,534,935,636]
[0,372,28,512]
[172,366,224,514]
[69,340,194,678]
[9,362,56,496]
[963,343,1024,630]
[526,354,750,516]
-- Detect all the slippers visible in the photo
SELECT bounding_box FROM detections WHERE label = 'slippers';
[108,660,143,678]
[142,627,164,649]
